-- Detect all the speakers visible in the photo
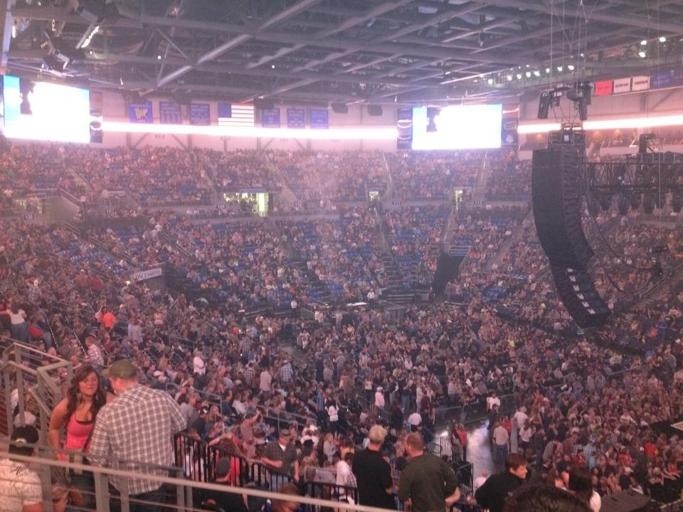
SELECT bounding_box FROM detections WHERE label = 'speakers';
[530,129,611,328]
[331,104,349,114]
[368,105,383,116]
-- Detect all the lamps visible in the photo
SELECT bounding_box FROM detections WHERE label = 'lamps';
[17,78,35,114]
[174,94,191,106]
[126,90,141,104]
[425,107,440,132]
[254,98,273,110]
[367,104,383,116]
[332,104,348,112]
[583,149,683,217]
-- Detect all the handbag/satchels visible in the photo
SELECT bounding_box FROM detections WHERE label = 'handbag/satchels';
[46,451,85,485]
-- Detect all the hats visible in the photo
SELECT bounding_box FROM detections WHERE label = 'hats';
[280,428,292,438]
[9,425,38,454]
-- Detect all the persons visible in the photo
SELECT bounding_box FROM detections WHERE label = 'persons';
[1,134,682,512]
